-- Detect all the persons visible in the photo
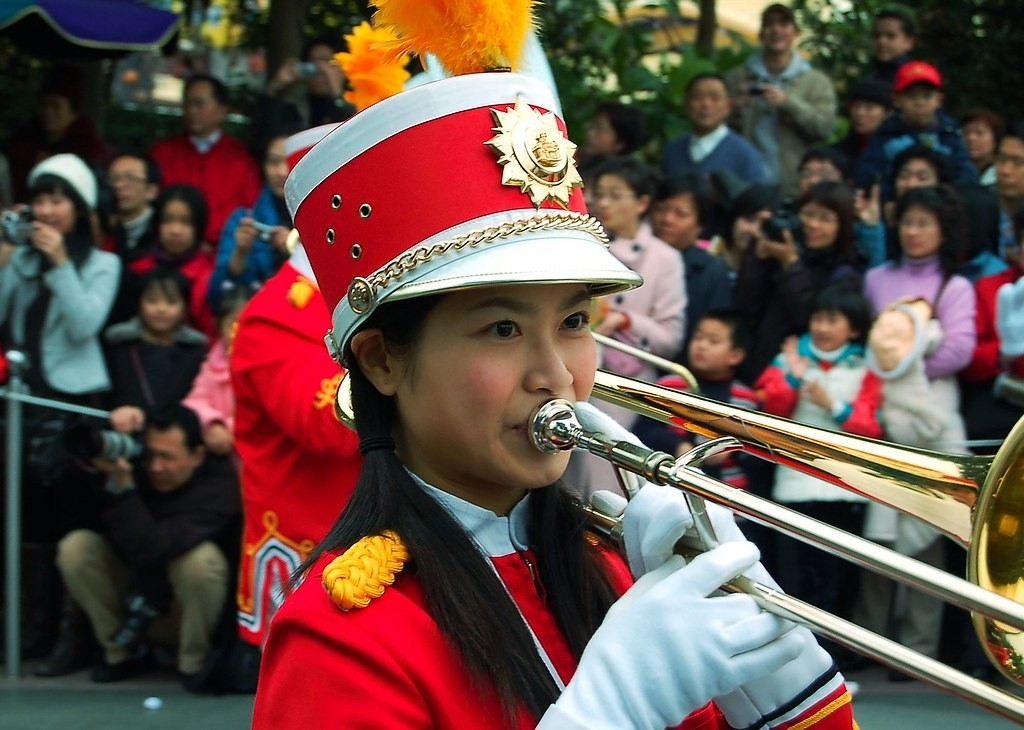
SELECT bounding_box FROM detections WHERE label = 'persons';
[579,4,1024,683]
[0,33,356,700]
[224,67,864,729]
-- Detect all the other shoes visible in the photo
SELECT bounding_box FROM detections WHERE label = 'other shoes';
[91,657,134,683]
[31,636,101,677]
[835,652,876,673]
[184,672,205,692]
[888,670,916,683]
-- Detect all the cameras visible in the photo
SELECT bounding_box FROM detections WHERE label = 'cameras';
[0,205,38,247]
[81,430,148,463]
[762,209,801,242]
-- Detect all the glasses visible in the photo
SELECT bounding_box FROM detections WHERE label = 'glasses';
[109,174,145,184]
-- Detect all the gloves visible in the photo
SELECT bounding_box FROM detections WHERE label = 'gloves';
[590,466,785,595]
[554,538,805,730]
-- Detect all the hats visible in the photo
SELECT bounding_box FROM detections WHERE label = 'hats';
[283,0,644,366]
[29,154,96,209]
[896,63,943,91]
[762,5,798,29]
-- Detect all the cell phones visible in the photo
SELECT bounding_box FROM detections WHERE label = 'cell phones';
[255,222,273,241]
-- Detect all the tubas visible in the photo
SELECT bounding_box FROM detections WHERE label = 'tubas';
[335,329,1023,724]
